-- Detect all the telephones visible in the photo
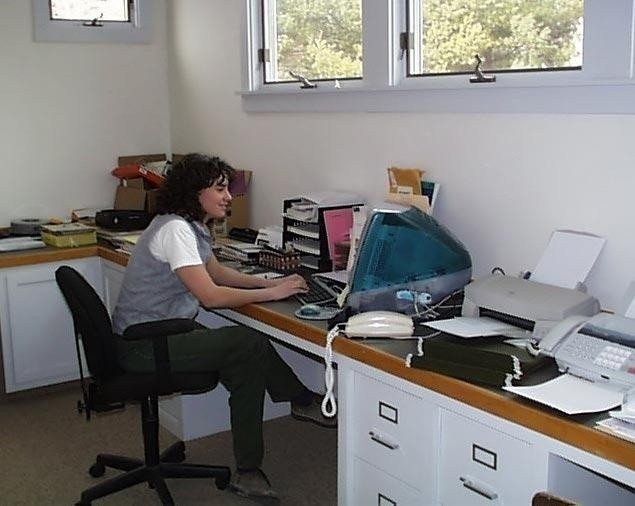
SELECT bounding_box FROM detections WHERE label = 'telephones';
[345,311,414,337]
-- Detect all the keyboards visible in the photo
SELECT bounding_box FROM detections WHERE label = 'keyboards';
[294,278,337,305]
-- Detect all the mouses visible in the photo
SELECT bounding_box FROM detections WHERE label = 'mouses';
[301,304,320,315]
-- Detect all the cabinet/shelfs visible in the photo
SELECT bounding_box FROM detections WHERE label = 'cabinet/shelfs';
[281,188,365,272]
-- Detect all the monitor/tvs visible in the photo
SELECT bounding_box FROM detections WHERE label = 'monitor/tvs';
[345,200,472,314]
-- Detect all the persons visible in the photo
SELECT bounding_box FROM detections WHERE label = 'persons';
[114,154,338,506]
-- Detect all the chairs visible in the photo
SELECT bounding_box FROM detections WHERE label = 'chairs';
[53,263,231,505]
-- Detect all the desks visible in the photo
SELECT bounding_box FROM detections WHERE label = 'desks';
[1,218,634,502]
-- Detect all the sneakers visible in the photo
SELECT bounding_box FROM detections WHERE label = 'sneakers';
[230,469,280,505]
[291,392,338,428]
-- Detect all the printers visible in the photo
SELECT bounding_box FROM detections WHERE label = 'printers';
[453,275,600,340]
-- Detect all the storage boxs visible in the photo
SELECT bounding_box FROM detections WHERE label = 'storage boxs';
[113,150,166,217]
[172,166,256,237]
